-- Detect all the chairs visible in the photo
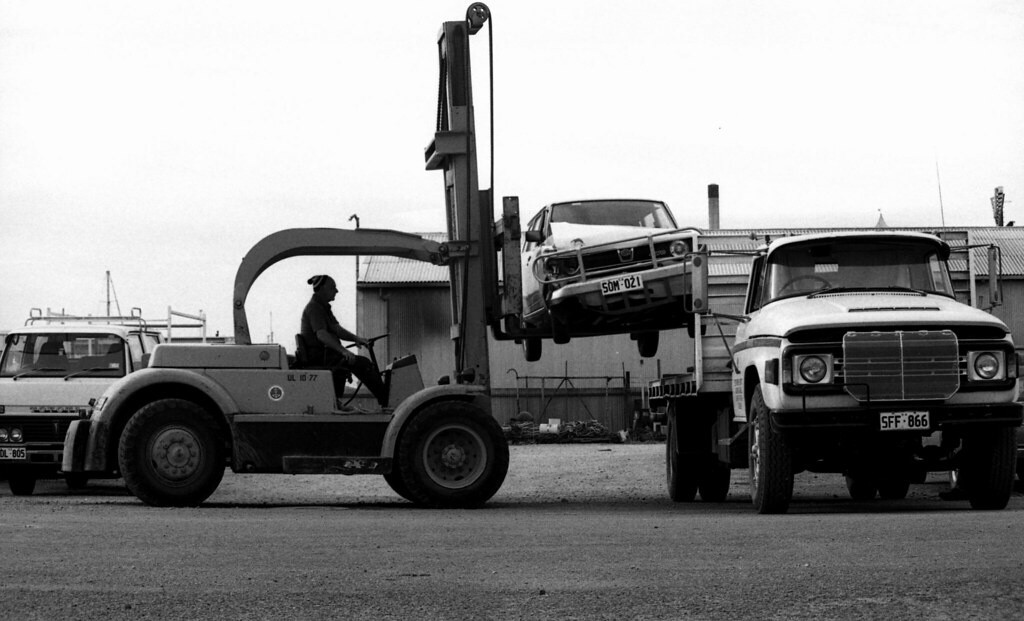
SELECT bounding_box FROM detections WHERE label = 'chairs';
[291,333,349,399]
[34,342,71,372]
[104,343,124,369]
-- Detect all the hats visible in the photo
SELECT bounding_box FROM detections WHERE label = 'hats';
[308,275,328,292]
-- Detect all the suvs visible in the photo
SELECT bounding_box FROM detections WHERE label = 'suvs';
[504,198,709,362]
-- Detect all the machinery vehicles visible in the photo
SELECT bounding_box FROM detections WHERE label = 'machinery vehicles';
[52,0,714,510]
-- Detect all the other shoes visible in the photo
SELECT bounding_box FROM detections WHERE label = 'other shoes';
[939,487,963,500]
[337,401,354,411]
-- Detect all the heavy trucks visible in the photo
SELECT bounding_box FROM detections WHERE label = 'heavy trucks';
[664,229,1024,514]
[0,271,208,497]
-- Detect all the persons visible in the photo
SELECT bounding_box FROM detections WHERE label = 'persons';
[300,275,386,413]
